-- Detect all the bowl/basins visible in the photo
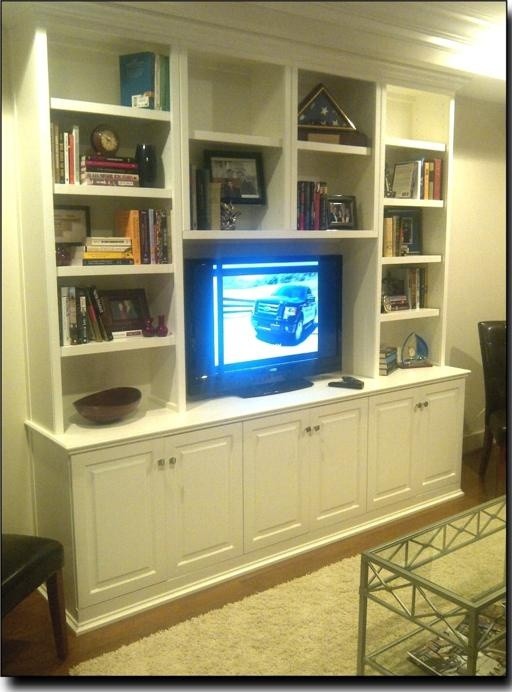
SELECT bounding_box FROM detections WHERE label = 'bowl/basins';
[72,386,142,423]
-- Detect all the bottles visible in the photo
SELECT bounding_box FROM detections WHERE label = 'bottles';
[135,143,156,187]
[143,313,167,337]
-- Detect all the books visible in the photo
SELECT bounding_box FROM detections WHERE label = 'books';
[297,181,330,231]
[380,157,443,376]
[50,51,169,347]
[190,164,223,231]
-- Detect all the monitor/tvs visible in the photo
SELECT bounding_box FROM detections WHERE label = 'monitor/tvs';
[184,254,342,398]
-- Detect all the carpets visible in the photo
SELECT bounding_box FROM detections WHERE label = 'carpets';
[67,496,508,678]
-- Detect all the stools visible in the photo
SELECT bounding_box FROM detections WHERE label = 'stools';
[0,532,70,660]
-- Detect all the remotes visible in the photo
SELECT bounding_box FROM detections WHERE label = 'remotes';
[328,381,362,389]
[342,375,364,386]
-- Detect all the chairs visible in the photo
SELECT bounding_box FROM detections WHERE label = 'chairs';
[478,319,507,481]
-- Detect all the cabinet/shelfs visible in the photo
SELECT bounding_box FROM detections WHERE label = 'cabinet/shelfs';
[385,82,454,377]
[188,50,286,231]
[46,28,183,432]
[369,380,468,514]
[67,421,240,612]
[241,398,370,556]
[296,68,376,232]
